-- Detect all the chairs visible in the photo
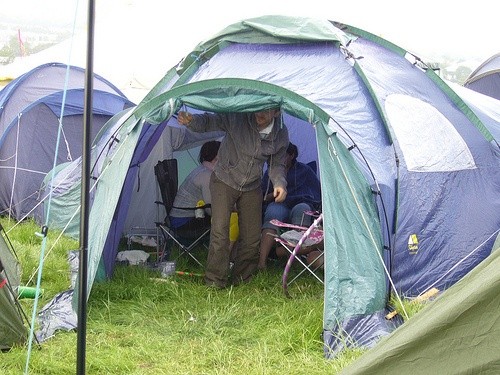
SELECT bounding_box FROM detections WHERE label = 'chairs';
[266,210,325,299]
[154,159,212,269]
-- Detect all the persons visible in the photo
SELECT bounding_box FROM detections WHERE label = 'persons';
[169,141,240,270]
[177,107,298,290]
[268,239,324,269]
[257,142,321,271]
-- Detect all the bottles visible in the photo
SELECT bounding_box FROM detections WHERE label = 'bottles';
[16,286,44,299]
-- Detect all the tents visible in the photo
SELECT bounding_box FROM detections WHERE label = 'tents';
[0,14,500,359]
[0,62,138,225]
[339,228,500,375]
[462,53,500,99]
[0,223,43,352]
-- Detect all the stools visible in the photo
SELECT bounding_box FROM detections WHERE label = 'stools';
[128,227,165,263]
